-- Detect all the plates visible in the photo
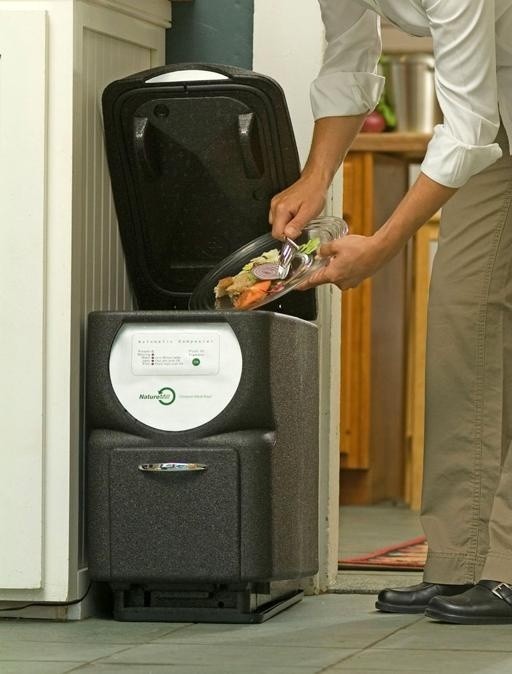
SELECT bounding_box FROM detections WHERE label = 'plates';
[188,216,349,312]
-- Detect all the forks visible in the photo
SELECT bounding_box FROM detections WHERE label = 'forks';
[279,234,297,269]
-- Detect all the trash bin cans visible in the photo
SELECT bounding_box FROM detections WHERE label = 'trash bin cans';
[88,63,318,623]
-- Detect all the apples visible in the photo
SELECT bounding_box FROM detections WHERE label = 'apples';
[360,112,386,133]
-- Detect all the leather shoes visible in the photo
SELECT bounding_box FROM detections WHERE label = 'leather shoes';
[375,580,512,624]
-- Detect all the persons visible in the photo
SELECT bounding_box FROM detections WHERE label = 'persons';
[268,0,512,625]
